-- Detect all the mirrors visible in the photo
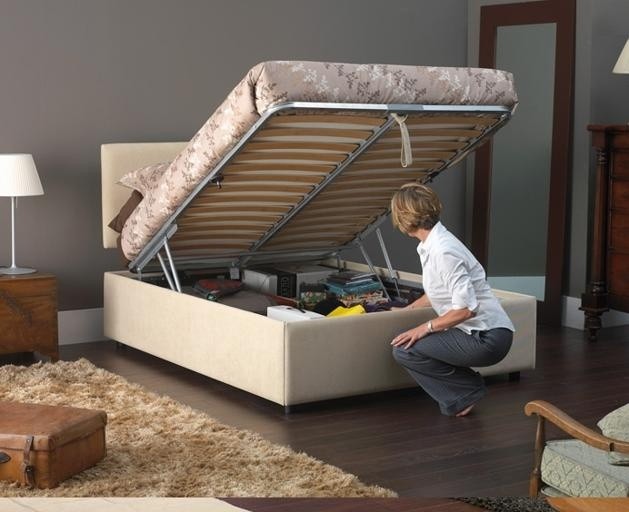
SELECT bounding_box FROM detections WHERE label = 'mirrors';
[469,0,577,328]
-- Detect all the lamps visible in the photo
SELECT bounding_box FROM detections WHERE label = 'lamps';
[611,34,629,74]
[1,150,46,275]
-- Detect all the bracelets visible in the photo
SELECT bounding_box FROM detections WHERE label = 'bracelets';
[428,318,433,333]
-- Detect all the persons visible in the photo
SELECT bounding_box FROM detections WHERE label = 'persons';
[389,182,512,416]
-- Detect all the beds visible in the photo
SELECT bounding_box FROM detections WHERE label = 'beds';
[99,58,536,416]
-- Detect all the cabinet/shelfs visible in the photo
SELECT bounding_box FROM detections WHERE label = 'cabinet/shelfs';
[577,123,629,344]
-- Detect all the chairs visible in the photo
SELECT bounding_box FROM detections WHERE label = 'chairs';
[524,401,629,497]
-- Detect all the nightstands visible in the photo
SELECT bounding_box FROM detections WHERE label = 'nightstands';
[0,273,59,363]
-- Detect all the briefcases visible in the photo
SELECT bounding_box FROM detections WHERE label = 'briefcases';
[0,402,107,490]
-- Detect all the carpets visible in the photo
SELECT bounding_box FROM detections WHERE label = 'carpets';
[0,356,399,497]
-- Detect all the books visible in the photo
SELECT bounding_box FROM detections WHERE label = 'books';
[329,270,375,287]
